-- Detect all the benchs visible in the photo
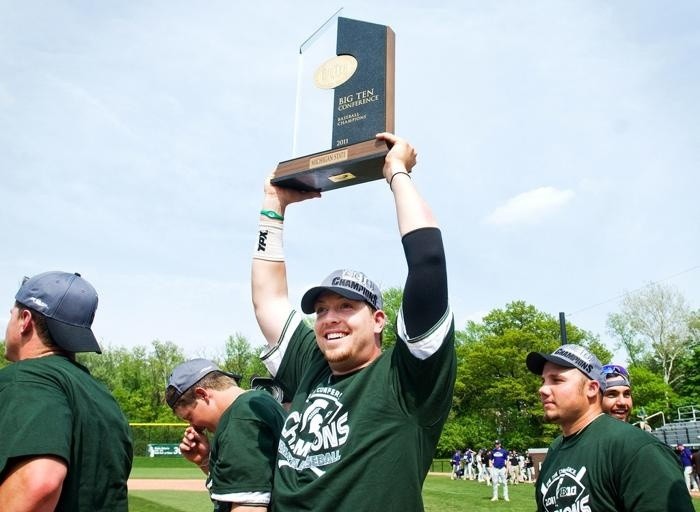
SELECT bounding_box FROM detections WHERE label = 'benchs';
[650,421,700,444]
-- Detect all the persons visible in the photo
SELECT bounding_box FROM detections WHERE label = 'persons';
[446,438,700,502]
[0,270,133,512]
[250,131,457,512]
[599,365,632,423]
[162,356,289,512]
[524,343,695,511]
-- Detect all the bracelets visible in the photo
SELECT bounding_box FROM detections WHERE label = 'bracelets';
[389,171,412,190]
[258,209,285,223]
[201,452,212,469]
[253,224,290,264]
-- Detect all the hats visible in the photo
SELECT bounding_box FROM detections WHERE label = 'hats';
[494,439,501,444]
[599,364,631,388]
[525,343,609,396]
[12,269,104,355]
[299,268,384,315]
[164,357,244,408]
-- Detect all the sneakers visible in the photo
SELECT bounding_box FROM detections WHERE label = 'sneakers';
[503,497,511,502]
[490,495,499,502]
[446,475,539,486]
